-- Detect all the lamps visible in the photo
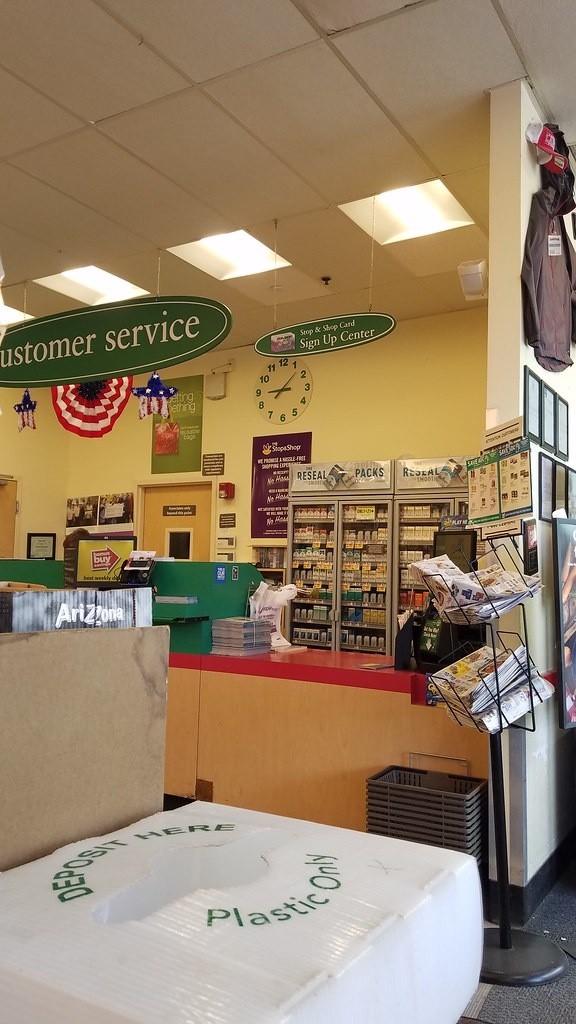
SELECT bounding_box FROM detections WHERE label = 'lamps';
[456,258,489,302]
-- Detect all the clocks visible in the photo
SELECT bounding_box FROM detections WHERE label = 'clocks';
[254,357,313,426]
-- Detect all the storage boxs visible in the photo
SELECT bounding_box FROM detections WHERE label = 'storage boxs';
[0,801,484,1024]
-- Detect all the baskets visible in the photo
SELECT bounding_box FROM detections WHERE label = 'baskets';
[366,765,488,867]
